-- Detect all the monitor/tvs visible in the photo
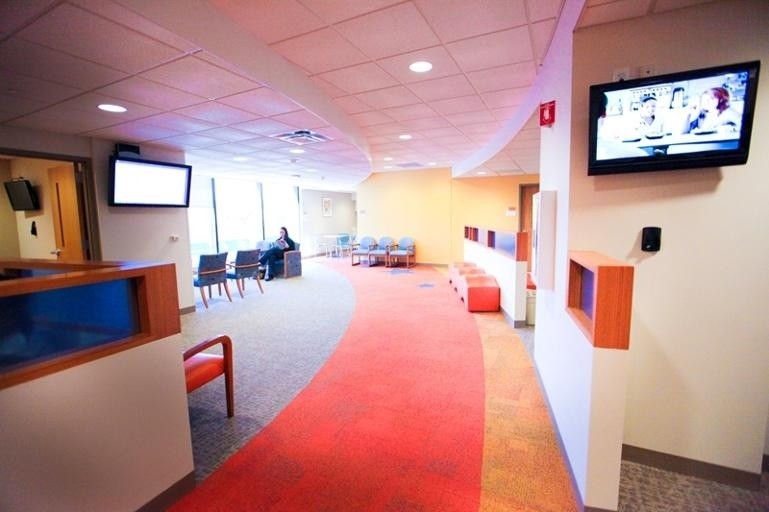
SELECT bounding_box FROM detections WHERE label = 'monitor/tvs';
[3,180,37,211]
[588,58,763,177]
[104,153,192,208]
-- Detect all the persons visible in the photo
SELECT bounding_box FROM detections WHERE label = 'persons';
[596,86,742,161]
[257,226,295,281]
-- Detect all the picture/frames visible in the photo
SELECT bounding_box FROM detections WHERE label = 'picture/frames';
[323,199,332,217]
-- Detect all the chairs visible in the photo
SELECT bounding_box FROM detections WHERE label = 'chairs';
[256,241,301,279]
[314,233,416,268]
[193,251,232,308]
[226,248,264,298]
[182,335,234,418]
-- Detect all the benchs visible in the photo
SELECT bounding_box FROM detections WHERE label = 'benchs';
[526,272,536,325]
[448,262,500,313]
